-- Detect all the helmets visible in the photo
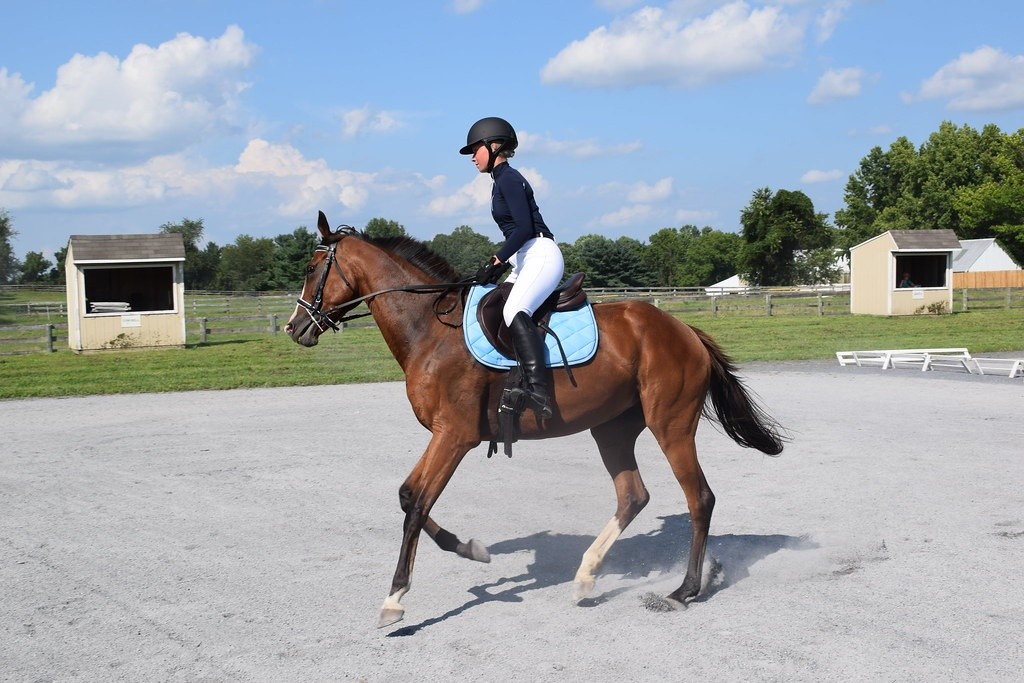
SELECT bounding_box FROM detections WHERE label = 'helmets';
[459,117,518,155]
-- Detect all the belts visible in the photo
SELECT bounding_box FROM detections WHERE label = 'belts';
[529,232,555,241]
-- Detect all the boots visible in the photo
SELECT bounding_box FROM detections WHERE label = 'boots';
[510,311,553,419]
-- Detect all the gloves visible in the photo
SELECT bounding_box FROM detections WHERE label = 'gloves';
[490,263,510,284]
[476,261,494,286]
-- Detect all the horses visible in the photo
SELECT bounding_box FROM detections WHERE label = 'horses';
[284,210,803,629]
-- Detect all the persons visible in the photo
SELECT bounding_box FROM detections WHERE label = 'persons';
[899,273,916,288]
[461,118,566,421]
